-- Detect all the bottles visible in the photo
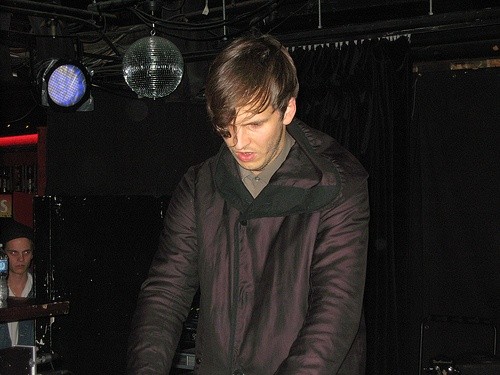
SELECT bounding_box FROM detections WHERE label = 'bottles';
[0,244,8,308]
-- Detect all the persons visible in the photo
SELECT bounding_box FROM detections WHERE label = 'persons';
[126,35,369,375]
[0,226,35,349]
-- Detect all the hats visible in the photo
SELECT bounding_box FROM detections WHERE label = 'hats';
[0,217,34,244]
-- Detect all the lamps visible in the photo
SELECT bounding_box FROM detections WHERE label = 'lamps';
[26,36,95,112]
